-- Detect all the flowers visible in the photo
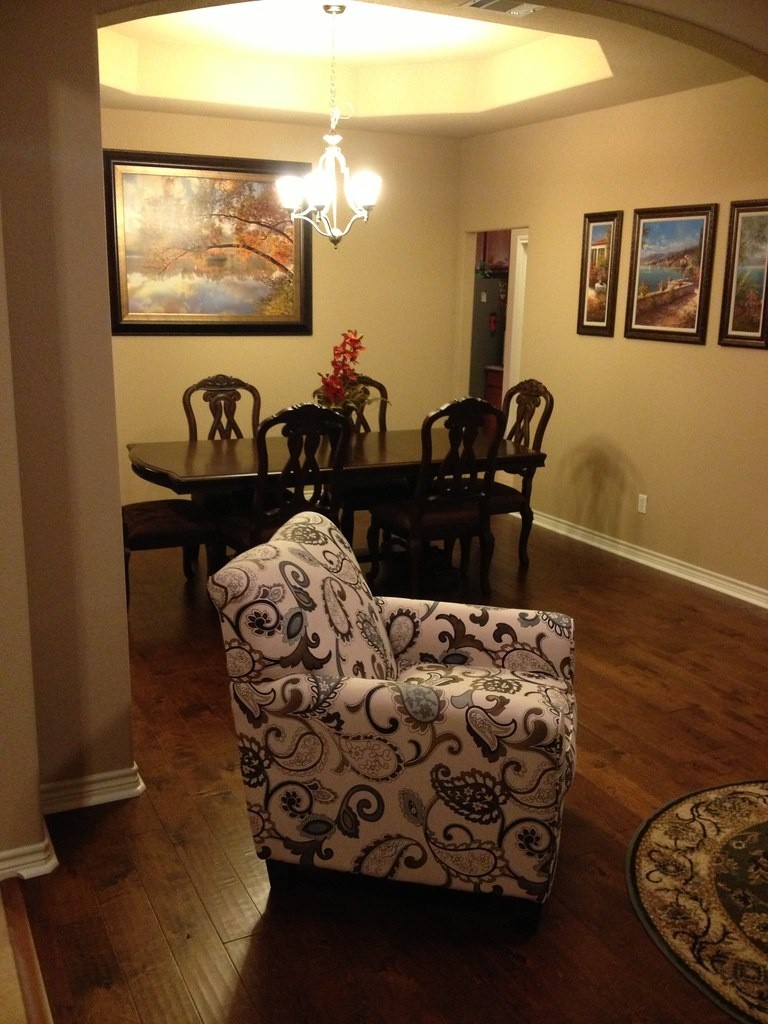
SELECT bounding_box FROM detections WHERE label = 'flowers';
[313,329,393,412]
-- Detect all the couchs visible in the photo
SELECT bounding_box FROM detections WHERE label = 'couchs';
[207,512,578,907]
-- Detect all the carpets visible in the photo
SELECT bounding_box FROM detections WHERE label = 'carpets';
[623,779,768,1024]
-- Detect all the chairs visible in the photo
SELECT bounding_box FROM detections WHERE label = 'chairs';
[312,373,397,571]
[321,405,355,466]
[202,400,350,570]
[427,378,556,582]
[186,374,263,565]
[123,497,203,618]
[385,395,508,588]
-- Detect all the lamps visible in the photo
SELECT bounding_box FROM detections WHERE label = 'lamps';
[271,2,382,251]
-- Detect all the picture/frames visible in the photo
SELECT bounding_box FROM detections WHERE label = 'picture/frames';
[718,197,767,349]
[102,148,315,338]
[577,209,622,338]
[623,201,717,346]
